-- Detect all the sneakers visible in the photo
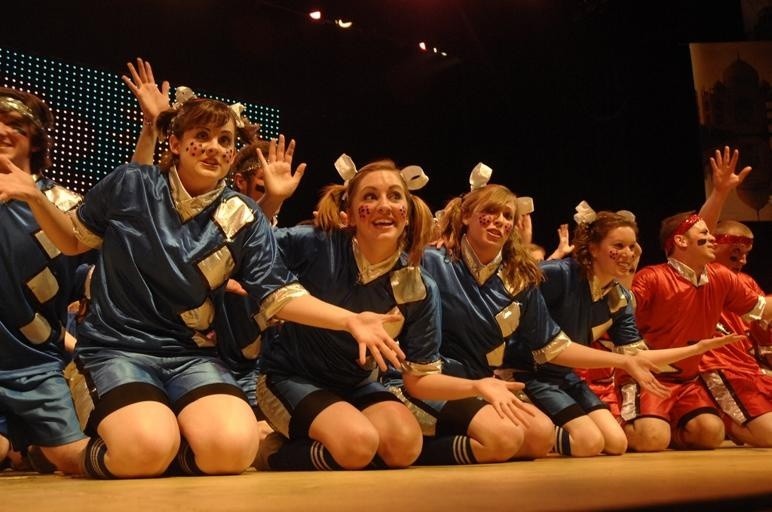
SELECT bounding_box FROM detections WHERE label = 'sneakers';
[253,432,287,472]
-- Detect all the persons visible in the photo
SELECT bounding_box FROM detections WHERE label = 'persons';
[2,56,772,479]
